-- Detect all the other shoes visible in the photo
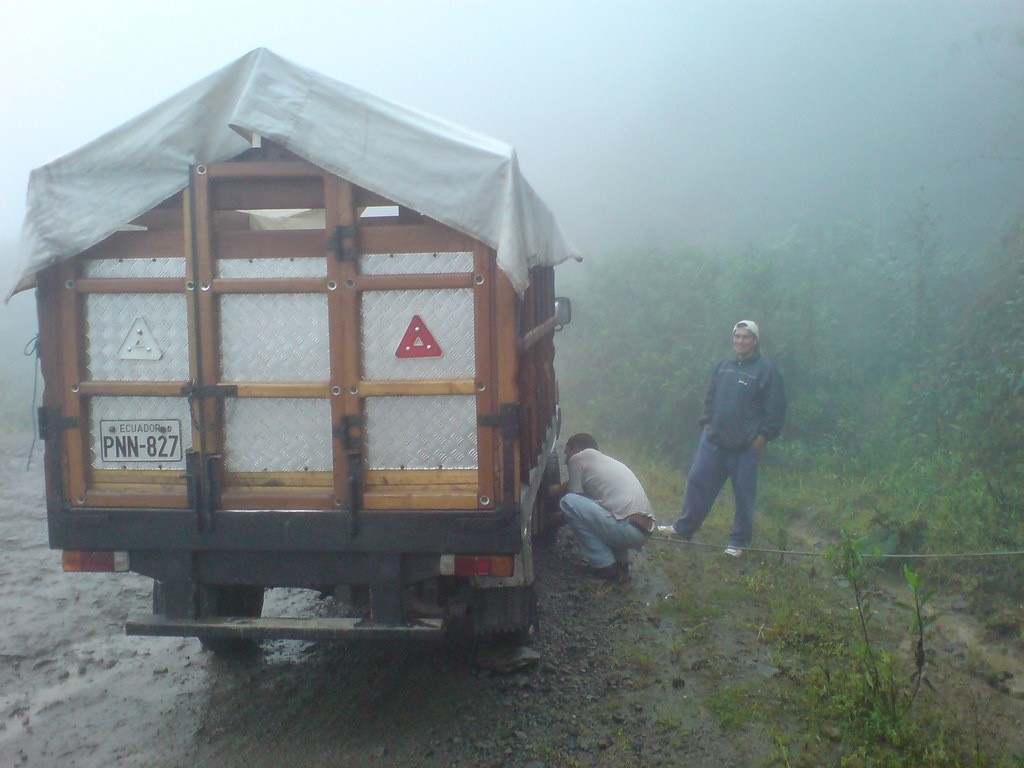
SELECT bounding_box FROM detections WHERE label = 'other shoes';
[725,545,744,558]
[658,524,679,537]
[592,562,618,579]
[619,562,629,575]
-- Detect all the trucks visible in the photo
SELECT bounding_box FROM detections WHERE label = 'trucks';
[1,46,573,654]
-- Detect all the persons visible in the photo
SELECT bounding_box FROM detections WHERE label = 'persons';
[657,320,787,559]
[538,433,655,578]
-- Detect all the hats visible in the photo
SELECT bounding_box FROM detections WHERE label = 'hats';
[733,320,759,339]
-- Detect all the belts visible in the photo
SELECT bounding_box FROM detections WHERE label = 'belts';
[625,518,649,534]
